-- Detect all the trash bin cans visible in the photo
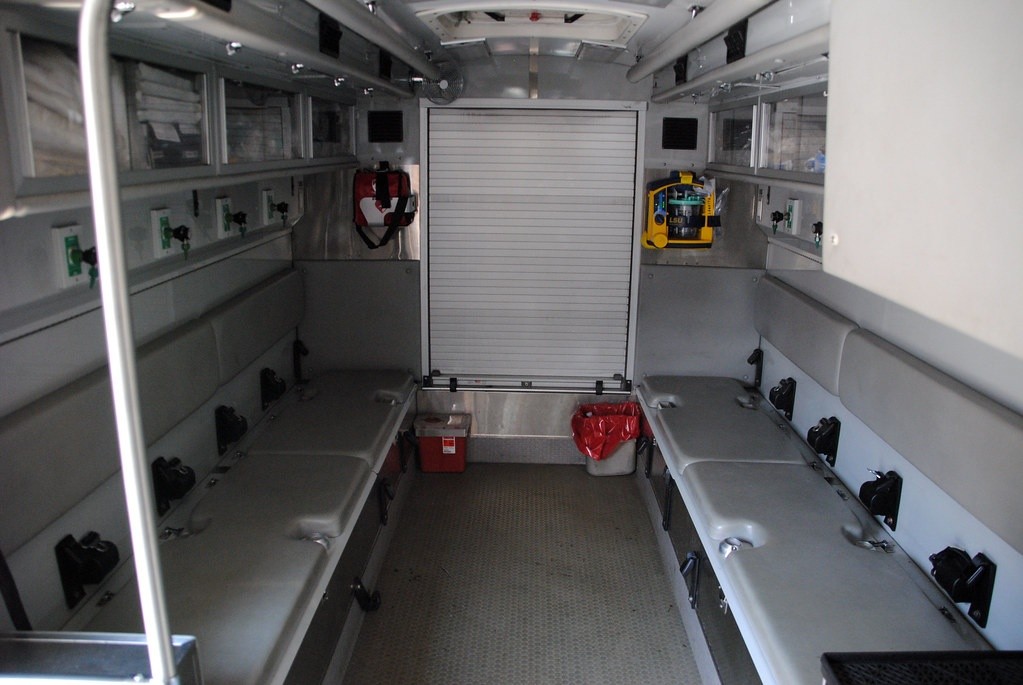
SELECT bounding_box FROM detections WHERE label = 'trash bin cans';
[414,414,471,472]
[571,402,640,476]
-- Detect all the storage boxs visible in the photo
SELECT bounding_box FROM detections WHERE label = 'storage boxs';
[415,413,471,472]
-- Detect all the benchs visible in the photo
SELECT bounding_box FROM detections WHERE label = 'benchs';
[0,258,421,685]
[635,274,1023,685]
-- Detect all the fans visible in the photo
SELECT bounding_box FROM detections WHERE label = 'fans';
[409,57,464,106]
